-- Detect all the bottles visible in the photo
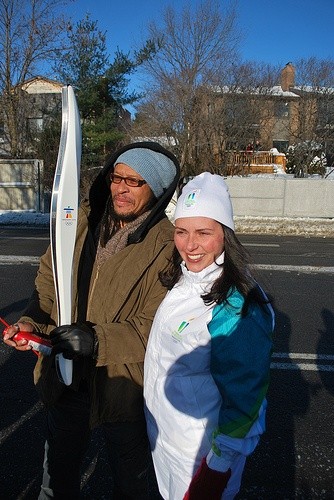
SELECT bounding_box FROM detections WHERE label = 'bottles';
[4,328,53,355]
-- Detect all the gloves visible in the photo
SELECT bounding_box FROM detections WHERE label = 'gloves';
[49,323,99,360]
[184,457,231,500]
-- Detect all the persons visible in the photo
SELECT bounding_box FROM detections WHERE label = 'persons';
[142,171,275,500]
[3,141,181,500]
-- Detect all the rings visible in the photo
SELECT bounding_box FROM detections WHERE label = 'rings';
[13,323,20,332]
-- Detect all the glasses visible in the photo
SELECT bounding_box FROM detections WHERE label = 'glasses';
[110,173,145,187]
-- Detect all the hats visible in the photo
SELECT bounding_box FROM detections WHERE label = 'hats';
[114,148,177,198]
[174,172,235,232]
